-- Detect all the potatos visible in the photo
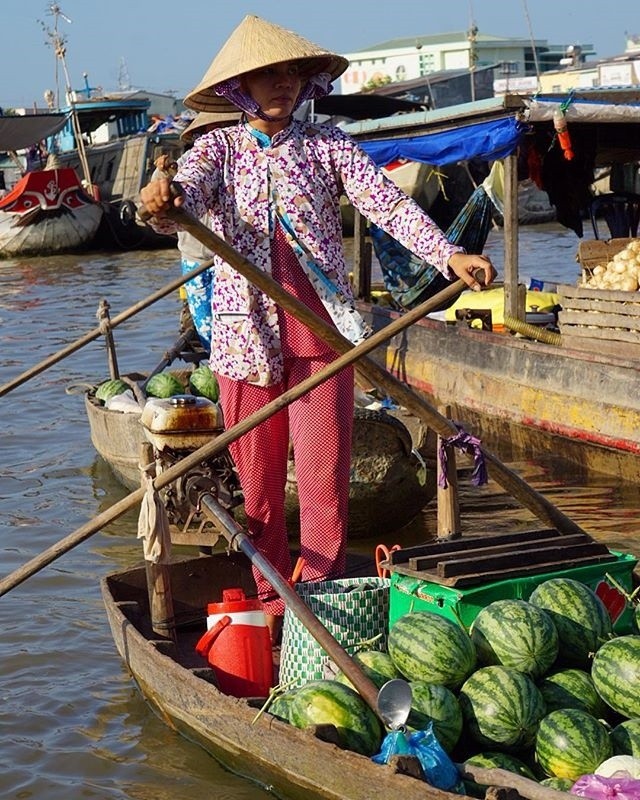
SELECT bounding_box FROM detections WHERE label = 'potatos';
[567,239,640,333]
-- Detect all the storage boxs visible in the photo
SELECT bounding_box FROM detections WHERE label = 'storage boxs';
[387,549,639,636]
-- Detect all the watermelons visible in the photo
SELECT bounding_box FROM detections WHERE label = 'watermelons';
[190,366,220,404]
[96,379,131,401]
[252,572,640,792]
[146,373,185,399]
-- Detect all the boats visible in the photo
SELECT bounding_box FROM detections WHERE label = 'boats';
[0,0,193,255]
[331,106,534,246]
[98,541,520,800]
[62,297,428,501]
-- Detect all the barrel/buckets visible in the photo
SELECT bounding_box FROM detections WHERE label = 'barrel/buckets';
[141,395,224,433]
[526,312,554,333]
[196,589,274,698]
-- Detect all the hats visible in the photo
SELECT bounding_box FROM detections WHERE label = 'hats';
[179,112,242,145]
[183,16,350,114]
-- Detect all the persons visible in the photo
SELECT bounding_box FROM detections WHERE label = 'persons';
[139,15,498,645]
[150,156,172,183]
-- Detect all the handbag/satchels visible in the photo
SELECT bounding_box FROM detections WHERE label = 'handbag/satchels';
[279,542,400,689]
